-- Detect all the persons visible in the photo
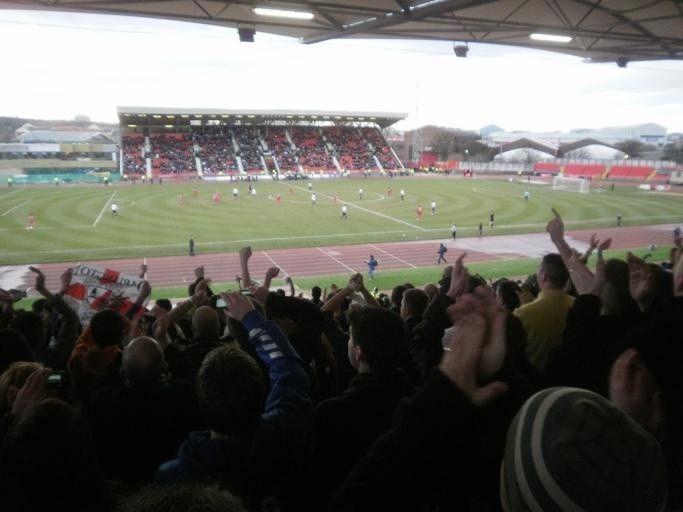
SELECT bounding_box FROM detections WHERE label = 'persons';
[357,186,437,221]
[195,180,348,219]
[0,209,683,512]
[1,127,474,185]
[112,203,120,216]
[509,170,531,200]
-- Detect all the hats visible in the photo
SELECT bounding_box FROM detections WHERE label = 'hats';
[504,386,669,511]
